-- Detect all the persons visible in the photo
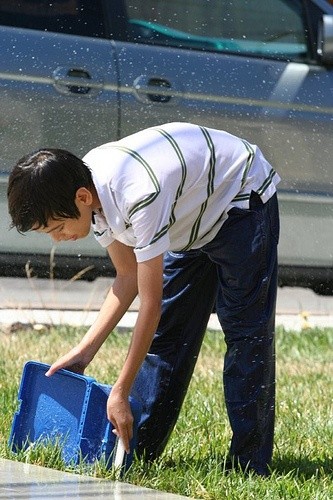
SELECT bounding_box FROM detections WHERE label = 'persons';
[6,121,281,483]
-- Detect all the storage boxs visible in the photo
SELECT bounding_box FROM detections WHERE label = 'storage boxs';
[8,360,141,477]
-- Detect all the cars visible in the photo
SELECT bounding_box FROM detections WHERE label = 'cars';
[0,0,333,295]
